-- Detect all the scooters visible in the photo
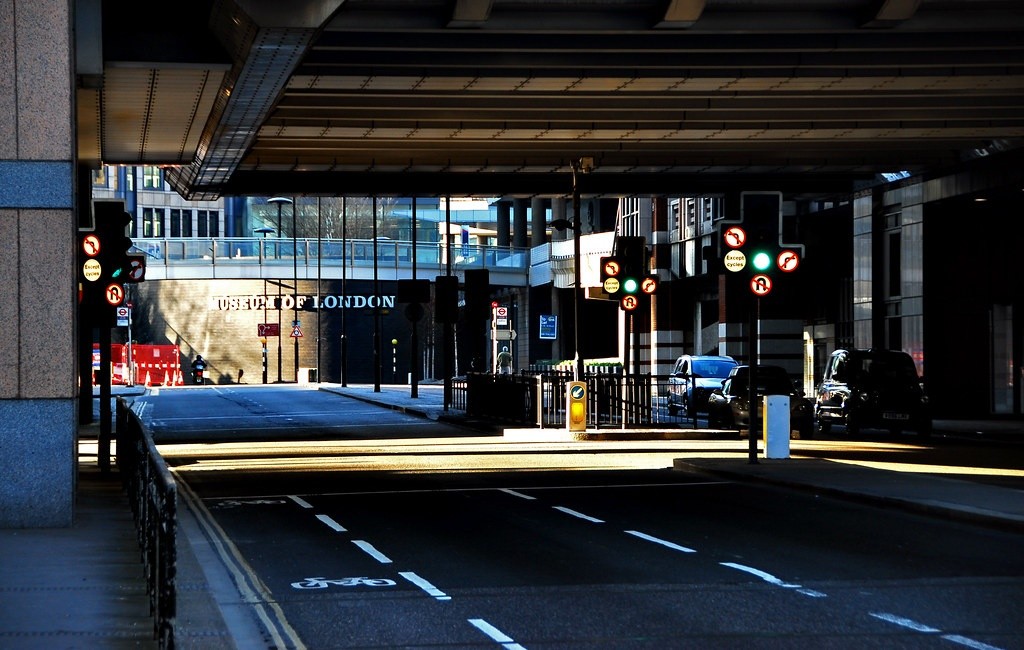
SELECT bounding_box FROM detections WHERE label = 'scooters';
[191,360,207,385]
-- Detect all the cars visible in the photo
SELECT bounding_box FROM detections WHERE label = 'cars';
[709,364,814,440]
[817,347,932,439]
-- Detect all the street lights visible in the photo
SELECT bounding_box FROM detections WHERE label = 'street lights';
[254,227,276,260]
[267,198,292,260]
[548,159,586,383]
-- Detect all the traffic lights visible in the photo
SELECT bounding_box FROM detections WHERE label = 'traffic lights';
[92,198,132,282]
[490,299,501,321]
[615,234,645,297]
[743,191,783,298]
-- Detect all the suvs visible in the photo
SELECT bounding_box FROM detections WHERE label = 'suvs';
[666,353,744,419]
